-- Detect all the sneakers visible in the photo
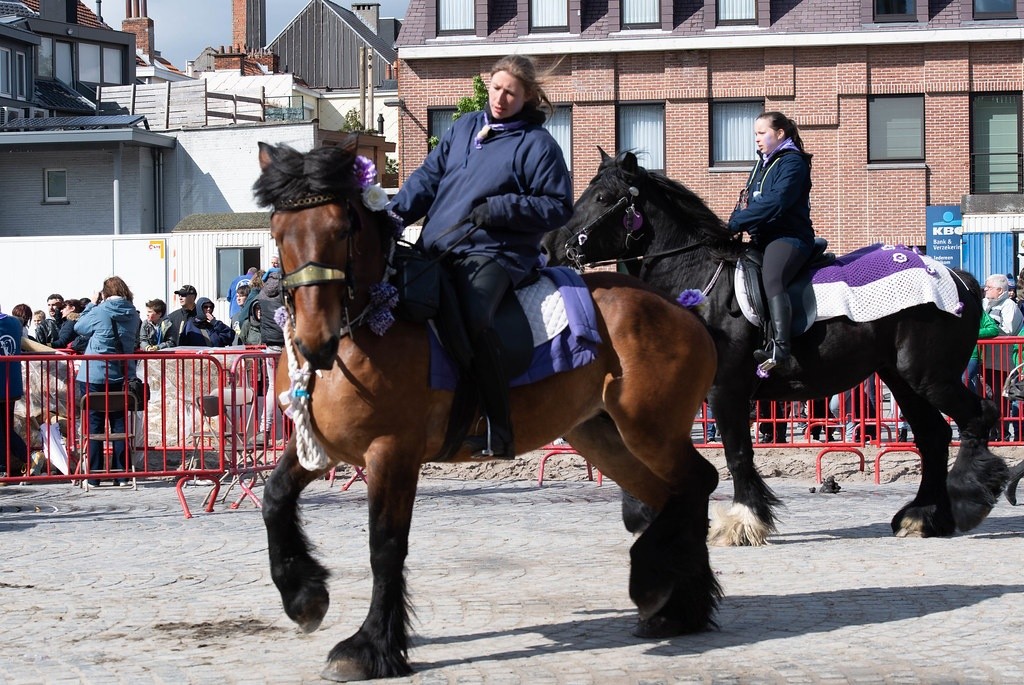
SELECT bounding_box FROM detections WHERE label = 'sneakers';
[706,435,715,442]
[771,437,786,443]
[760,433,772,442]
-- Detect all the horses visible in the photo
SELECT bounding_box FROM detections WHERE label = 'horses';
[536,145,1009,550]
[251,130,790,684]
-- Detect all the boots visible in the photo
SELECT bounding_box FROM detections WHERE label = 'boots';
[459,326,516,461]
[898,429,907,442]
[754,293,791,376]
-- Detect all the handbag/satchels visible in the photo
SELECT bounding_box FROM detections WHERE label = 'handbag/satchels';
[121,375,150,411]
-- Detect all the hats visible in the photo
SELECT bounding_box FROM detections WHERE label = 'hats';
[174,285,197,299]
[1006,274,1017,291]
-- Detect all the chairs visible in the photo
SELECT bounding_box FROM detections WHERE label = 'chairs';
[184,386,256,487]
[79,390,138,492]
[196,393,271,506]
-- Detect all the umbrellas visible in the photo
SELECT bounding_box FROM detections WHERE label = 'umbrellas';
[38,422,68,475]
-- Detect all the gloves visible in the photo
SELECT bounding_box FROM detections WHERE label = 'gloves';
[469,201,491,230]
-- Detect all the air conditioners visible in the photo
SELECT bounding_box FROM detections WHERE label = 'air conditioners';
[0,106,23,131]
[21,106,49,130]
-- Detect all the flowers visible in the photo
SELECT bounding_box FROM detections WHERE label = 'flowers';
[354,155,393,212]
[677,289,706,307]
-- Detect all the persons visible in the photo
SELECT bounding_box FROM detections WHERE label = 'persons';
[0,304,27,487]
[133,285,236,352]
[12,294,94,351]
[728,111,814,377]
[1003,459,1024,506]
[961,268,1024,442]
[702,373,907,442]
[227,256,293,446]
[73,276,141,488]
[388,54,575,460]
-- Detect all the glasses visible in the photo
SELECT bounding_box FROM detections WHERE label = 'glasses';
[202,306,214,309]
[986,285,998,291]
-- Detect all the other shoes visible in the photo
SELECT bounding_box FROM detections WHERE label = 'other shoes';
[114,479,131,485]
[83,480,98,488]
[828,435,834,441]
[0,464,22,486]
[989,430,1011,441]
[813,436,819,440]
